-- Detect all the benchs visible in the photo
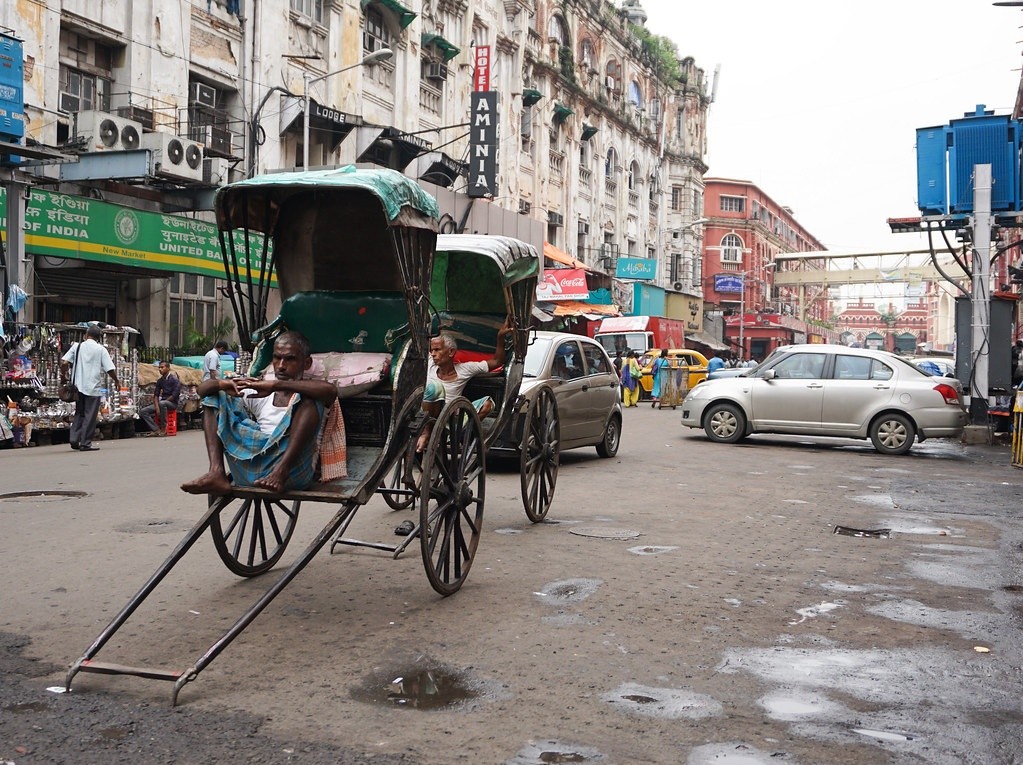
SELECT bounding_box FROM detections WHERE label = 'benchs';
[252,286,414,450]
[429,308,521,407]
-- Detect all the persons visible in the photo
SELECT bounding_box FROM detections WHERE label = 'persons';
[181,330,337,494]
[202,340,227,381]
[536,272,562,296]
[415,315,514,453]
[140,361,181,436]
[707,352,758,374]
[61,326,121,451]
[613,349,668,408]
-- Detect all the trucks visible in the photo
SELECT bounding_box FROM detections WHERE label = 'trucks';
[591,316,686,373]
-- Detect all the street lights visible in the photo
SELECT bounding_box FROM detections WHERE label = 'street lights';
[302,48,394,171]
[739,262,777,360]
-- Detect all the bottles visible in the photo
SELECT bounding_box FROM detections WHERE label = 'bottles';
[143,344,192,362]
[119,366,132,406]
[12,355,36,377]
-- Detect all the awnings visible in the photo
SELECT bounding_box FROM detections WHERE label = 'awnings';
[360,0,419,35]
[685,328,731,350]
[544,241,608,276]
[280,94,362,152]
[524,90,542,107]
[552,103,574,123]
[356,125,432,172]
[581,121,597,141]
[405,151,468,189]
[532,288,622,317]
[422,33,461,63]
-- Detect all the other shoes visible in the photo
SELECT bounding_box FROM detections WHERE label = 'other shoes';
[80,445,99,450]
[149,430,161,436]
[71,443,79,449]
[158,430,167,436]
[394,520,414,534]
[416,525,432,538]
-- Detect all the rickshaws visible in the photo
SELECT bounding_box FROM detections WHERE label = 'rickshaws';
[65,162,561,711]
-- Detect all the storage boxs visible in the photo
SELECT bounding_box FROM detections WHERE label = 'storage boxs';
[153,410,177,436]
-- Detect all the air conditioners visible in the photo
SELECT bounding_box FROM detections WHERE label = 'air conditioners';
[142,132,204,185]
[521,200,530,213]
[194,126,234,156]
[549,212,564,227]
[425,62,449,82]
[601,244,611,258]
[188,81,216,109]
[579,221,589,235]
[673,280,692,292]
[204,156,232,186]
[67,109,144,152]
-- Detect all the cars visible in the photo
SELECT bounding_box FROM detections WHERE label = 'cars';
[681,343,967,455]
[445,331,624,459]
[618,349,711,403]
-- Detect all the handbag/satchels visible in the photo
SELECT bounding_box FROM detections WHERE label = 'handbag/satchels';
[651,359,658,375]
[631,360,642,379]
[58,382,79,403]
